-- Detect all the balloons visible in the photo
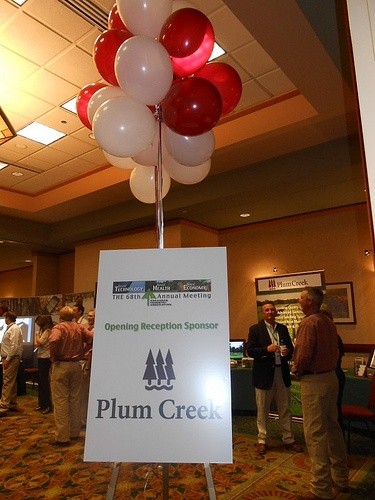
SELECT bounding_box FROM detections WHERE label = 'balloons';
[76,0,242,203]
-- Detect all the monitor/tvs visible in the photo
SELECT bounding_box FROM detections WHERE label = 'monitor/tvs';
[0,316,35,346]
[230,339,246,360]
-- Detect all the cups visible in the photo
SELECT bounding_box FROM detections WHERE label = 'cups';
[279,345,286,356]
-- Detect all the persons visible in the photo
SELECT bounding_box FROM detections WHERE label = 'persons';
[290,287,350,500]
[0,304,96,446]
[246,302,303,453]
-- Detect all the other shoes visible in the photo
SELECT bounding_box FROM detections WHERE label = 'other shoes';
[8,407,17,411]
[285,443,303,452]
[332,478,348,492]
[258,444,267,454]
[297,491,336,500]
[70,436,79,441]
[0,413,6,417]
[48,438,71,445]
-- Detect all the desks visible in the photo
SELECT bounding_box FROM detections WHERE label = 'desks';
[269,368,375,452]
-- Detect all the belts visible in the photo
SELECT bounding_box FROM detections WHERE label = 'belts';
[275,364,282,367]
[302,369,335,375]
[54,358,77,361]
[1,355,18,359]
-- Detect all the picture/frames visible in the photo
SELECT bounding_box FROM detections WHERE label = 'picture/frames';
[230,339,246,361]
[326,282,357,325]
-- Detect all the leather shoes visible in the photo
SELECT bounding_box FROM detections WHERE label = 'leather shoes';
[42,407,51,414]
[34,406,41,411]
